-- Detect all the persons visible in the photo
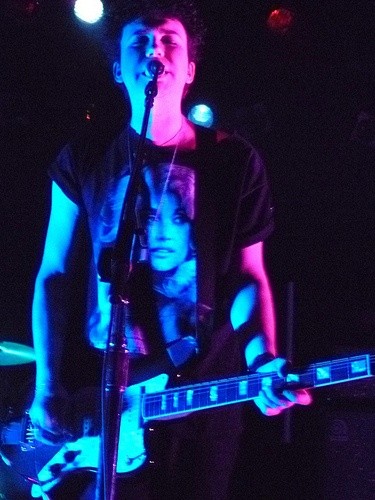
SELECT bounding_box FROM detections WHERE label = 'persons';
[26,1,312,500]
[138,163,197,341]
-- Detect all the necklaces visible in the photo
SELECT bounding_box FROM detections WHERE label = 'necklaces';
[127,120,185,261]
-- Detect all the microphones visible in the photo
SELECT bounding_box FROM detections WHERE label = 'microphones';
[145,60,165,79]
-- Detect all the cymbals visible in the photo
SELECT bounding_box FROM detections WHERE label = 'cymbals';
[0,342,35,366]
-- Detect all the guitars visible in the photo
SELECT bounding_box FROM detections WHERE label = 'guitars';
[0,353,375,500]
